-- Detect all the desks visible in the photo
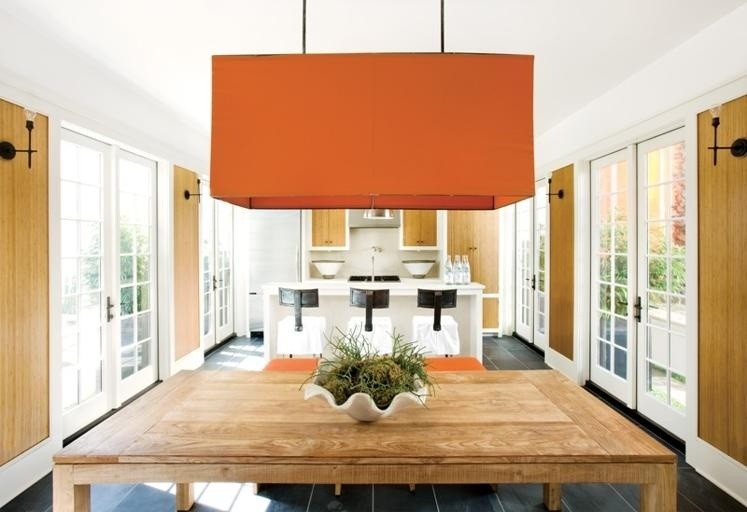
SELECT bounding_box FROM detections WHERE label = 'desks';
[261,282,487,366]
[50,363,681,512]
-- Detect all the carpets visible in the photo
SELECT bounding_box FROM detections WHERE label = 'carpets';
[261,355,486,373]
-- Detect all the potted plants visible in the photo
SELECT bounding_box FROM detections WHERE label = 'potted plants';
[300,323,435,427]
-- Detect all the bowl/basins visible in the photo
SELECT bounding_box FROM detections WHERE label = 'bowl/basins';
[402,259,437,279]
[311,260,345,279]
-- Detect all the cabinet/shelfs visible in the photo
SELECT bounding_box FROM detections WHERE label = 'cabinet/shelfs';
[312,209,346,247]
[445,209,503,339]
[403,209,437,248]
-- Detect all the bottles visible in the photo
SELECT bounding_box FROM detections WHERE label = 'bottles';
[444,253,471,286]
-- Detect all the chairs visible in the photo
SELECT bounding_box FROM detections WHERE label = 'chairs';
[276,287,464,359]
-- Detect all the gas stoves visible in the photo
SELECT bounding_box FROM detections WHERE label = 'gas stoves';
[349,275,400,282]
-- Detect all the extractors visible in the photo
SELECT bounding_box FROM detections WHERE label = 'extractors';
[349,209,401,229]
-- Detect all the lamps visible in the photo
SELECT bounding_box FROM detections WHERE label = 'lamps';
[546,175,564,203]
[184,176,202,203]
[707,103,746,167]
[206,0,540,217]
[0,105,38,170]
